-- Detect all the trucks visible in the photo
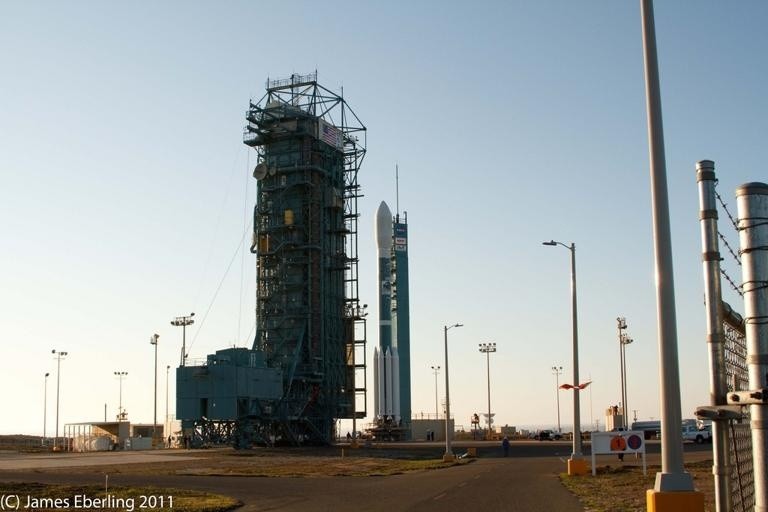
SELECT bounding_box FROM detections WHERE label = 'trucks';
[613,419,696,441]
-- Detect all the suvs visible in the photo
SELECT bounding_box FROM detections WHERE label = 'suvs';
[680,424,710,444]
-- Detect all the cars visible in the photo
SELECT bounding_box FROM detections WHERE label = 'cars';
[529,429,563,441]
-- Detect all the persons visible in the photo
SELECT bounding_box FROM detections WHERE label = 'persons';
[182,435,192,450]
[346,432,351,442]
[614,406,618,415]
[618,454,624,462]
[502,436,509,458]
[426,428,434,442]
[168,435,171,449]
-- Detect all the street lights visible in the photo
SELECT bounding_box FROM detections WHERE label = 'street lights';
[51,349,68,451]
[43,372,49,446]
[479,342,496,439]
[442,323,463,462]
[150,333,160,448]
[171,312,196,366]
[552,365,563,433]
[541,240,587,476]
[113,371,128,422]
[431,365,441,419]
[166,366,170,448]
[618,317,634,432]
